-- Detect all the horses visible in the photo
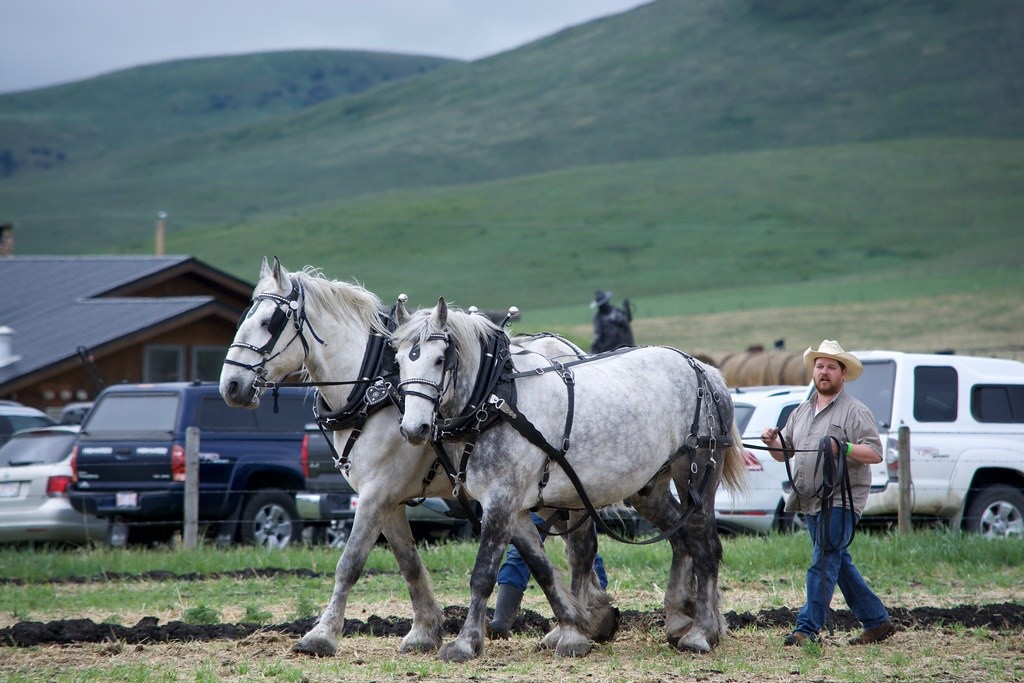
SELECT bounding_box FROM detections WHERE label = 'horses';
[218,255,762,664]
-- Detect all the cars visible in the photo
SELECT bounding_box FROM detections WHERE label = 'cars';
[0,400,108,543]
[601,384,807,536]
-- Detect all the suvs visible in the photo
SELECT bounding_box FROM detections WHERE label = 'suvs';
[68,378,468,547]
[806,349,1024,539]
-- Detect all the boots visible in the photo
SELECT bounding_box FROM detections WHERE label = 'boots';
[487,583,524,640]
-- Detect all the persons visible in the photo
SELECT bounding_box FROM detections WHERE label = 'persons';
[760,338,898,649]
[588,286,633,352]
[486,508,609,638]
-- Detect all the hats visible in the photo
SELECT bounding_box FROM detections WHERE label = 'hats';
[589,289,613,310]
[803,339,864,383]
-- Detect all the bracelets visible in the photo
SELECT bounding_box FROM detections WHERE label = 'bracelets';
[845,441,852,456]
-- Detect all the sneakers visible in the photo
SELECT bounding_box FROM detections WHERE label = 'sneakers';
[849,619,898,646]
[782,631,824,649]
